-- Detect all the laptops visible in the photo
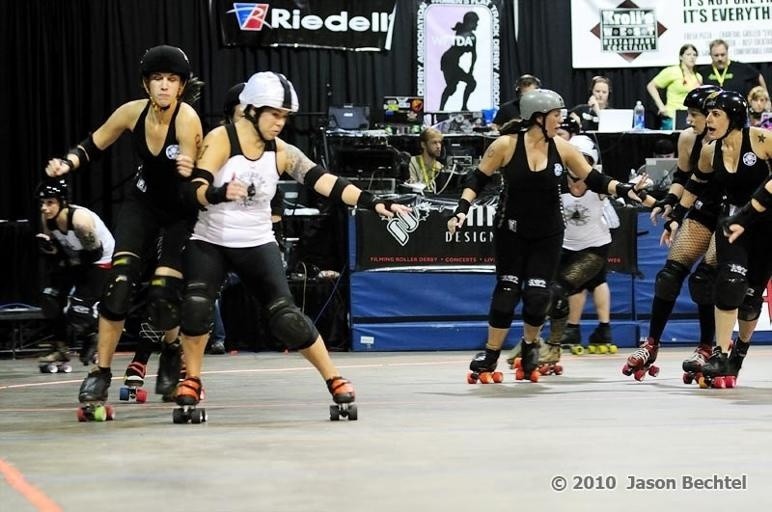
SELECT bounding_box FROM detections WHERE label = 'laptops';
[586,107,636,133]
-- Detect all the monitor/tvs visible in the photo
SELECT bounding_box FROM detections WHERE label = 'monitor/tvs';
[644,158,681,191]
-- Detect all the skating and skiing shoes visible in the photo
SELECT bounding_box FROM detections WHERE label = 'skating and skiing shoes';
[728,340,745,371]
[38,343,72,374]
[506,337,544,369]
[559,327,584,355]
[80,333,99,366]
[538,340,563,375]
[120,357,147,403]
[77,368,115,421]
[514,336,539,382]
[172,374,207,424]
[682,344,713,384]
[587,326,617,353]
[325,376,358,421]
[622,337,660,382]
[699,345,736,389]
[467,342,503,384]
[162,379,206,402]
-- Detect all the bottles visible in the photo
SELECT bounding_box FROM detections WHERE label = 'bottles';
[634,101,645,128]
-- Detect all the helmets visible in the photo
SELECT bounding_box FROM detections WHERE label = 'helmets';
[568,135,599,166]
[138,45,190,83]
[702,90,748,126]
[520,89,565,120]
[238,71,299,113]
[222,82,247,115]
[683,84,723,110]
[559,116,580,135]
[33,176,68,200]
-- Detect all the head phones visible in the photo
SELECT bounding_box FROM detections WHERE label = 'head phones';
[512,74,542,99]
[587,75,613,102]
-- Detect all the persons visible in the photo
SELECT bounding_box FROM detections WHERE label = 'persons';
[120,83,246,403]
[548,114,617,356]
[448,89,649,384]
[508,135,620,375]
[408,126,444,193]
[33,177,115,374]
[747,85,771,130]
[583,75,612,111]
[648,44,702,129]
[45,45,204,421]
[705,38,769,97]
[437,11,480,112]
[494,74,541,126]
[622,84,723,384]
[660,91,772,387]
[172,71,412,420]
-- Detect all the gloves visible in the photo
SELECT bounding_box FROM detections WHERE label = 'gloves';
[718,198,762,235]
[56,157,74,171]
[445,198,472,222]
[357,190,396,212]
[205,182,232,205]
[650,193,678,214]
[663,203,688,233]
[616,182,642,201]
